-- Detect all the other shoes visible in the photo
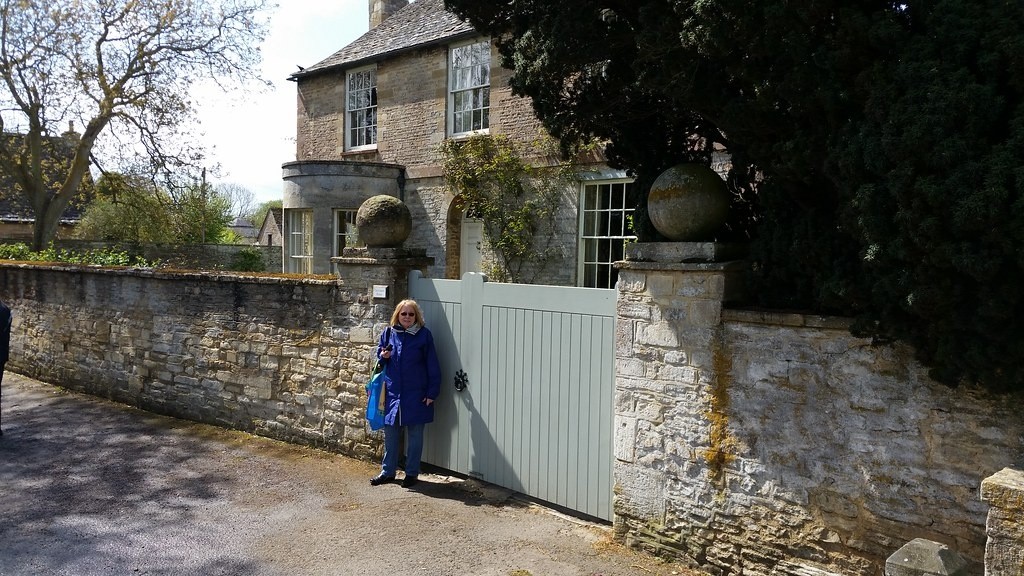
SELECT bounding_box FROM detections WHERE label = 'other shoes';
[0,428,3,437]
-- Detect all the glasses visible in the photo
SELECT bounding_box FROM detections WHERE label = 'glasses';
[399,311,416,317]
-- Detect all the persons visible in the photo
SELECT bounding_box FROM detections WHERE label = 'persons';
[0,303,13,437]
[366,299,442,486]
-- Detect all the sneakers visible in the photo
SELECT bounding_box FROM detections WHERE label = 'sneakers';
[400,473,418,488]
[370,473,396,486]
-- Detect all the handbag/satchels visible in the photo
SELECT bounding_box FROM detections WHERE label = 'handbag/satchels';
[364,360,386,431]
